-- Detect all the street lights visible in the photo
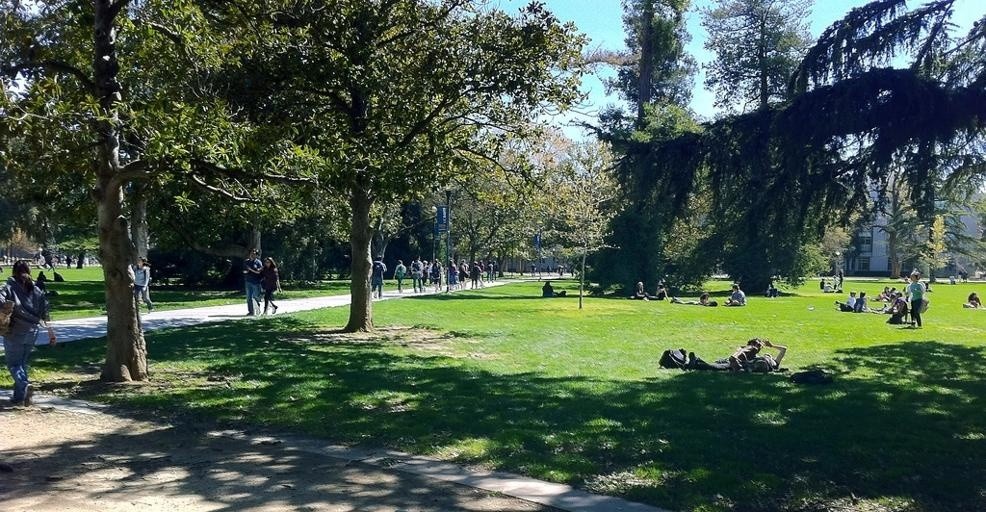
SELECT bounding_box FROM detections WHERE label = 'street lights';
[443,185,454,295]
[836,248,840,266]
[430,204,437,263]
[552,248,555,269]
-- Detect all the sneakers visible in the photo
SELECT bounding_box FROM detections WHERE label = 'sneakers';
[9,396,24,407]
[23,383,34,407]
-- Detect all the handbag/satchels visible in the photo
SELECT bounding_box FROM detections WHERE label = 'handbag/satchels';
[0,299,16,338]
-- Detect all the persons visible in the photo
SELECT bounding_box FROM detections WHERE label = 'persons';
[260,257,281,316]
[242,248,263,316]
[370,255,498,299]
[635,282,746,307]
[690,338,787,375]
[0,256,73,405]
[133,256,154,314]
[530,263,574,298]
[818,268,982,329]
